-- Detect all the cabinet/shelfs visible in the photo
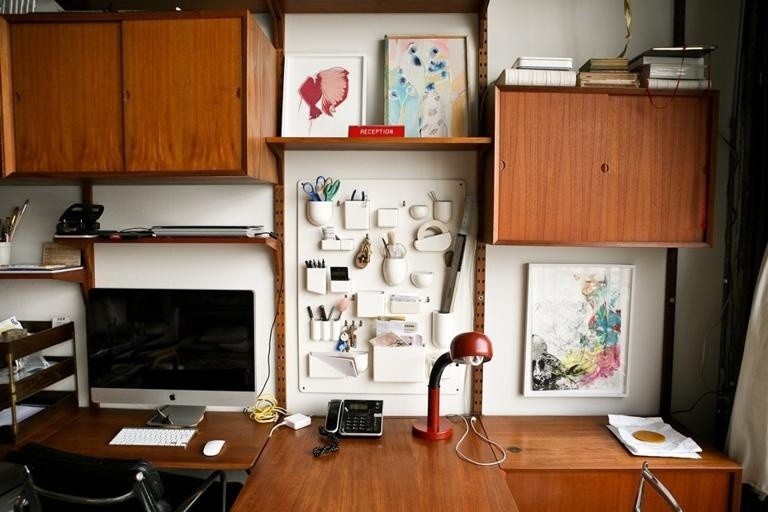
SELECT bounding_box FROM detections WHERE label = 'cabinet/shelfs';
[484,84,719,250]
[0,6,279,183]
[1,225,277,280]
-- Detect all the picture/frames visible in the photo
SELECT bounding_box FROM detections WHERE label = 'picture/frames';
[281,51,367,138]
[522,262,636,399]
[382,34,472,138]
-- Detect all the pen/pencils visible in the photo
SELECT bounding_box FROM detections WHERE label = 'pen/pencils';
[430,191,438,202]
[308,305,335,320]
[305,259,326,268]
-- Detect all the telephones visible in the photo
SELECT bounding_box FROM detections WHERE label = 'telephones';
[325,400,384,439]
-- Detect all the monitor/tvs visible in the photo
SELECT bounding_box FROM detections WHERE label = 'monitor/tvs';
[86,288,256,426]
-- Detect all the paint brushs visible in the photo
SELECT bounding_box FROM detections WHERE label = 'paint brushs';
[0,199,29,242]
[382,232,396,259]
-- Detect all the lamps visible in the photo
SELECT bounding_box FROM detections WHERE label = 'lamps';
[411,332,493,442]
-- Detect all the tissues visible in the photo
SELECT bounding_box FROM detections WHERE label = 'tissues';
[0,315,26,343]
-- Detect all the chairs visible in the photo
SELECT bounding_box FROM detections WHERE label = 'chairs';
[12,441,243,512]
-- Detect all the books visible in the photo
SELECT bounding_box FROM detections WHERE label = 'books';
[494,45,713,87]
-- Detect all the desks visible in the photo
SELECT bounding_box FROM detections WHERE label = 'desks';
[42,408,279,474]
[478,414,744,512]
[228,415,519,511]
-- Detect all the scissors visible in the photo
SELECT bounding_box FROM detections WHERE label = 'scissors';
[303,176,340,201]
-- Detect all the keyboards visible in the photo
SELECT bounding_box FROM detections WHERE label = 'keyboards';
[109,428,197,448]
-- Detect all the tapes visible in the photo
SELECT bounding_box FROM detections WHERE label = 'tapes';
[417,220,449,239]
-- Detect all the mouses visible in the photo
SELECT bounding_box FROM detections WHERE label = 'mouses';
[202,440,226,456]
